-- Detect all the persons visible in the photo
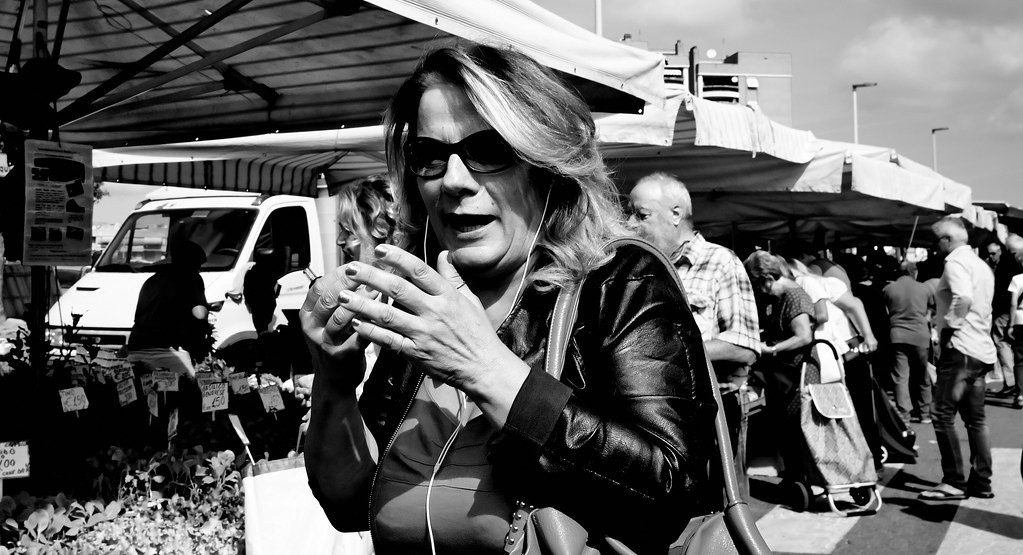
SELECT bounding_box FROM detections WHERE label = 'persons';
[299,46,1022,555]
[125,241,216,366]
[244,248,281,338]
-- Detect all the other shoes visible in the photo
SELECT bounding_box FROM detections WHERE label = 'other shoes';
[995,385,1019,399]
[834,487,879,516]
[1013,392,1023,409]
[909,410,932,423]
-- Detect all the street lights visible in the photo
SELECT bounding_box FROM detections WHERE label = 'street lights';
[931,127,949,171]
[851,81,879,145]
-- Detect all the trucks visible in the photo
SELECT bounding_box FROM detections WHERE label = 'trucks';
[48,171,357,398]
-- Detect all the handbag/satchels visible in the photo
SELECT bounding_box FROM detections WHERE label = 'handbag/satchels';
[505,232,774,555]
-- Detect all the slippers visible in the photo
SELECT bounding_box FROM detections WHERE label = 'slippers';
[968,488,995,498]
[920,488,969,500]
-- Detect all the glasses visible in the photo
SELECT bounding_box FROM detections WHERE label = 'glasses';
[401,127,523,179]
[937,236,950,242]
[987,252,996,256]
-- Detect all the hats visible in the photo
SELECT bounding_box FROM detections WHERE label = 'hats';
[171,239,210,266]
